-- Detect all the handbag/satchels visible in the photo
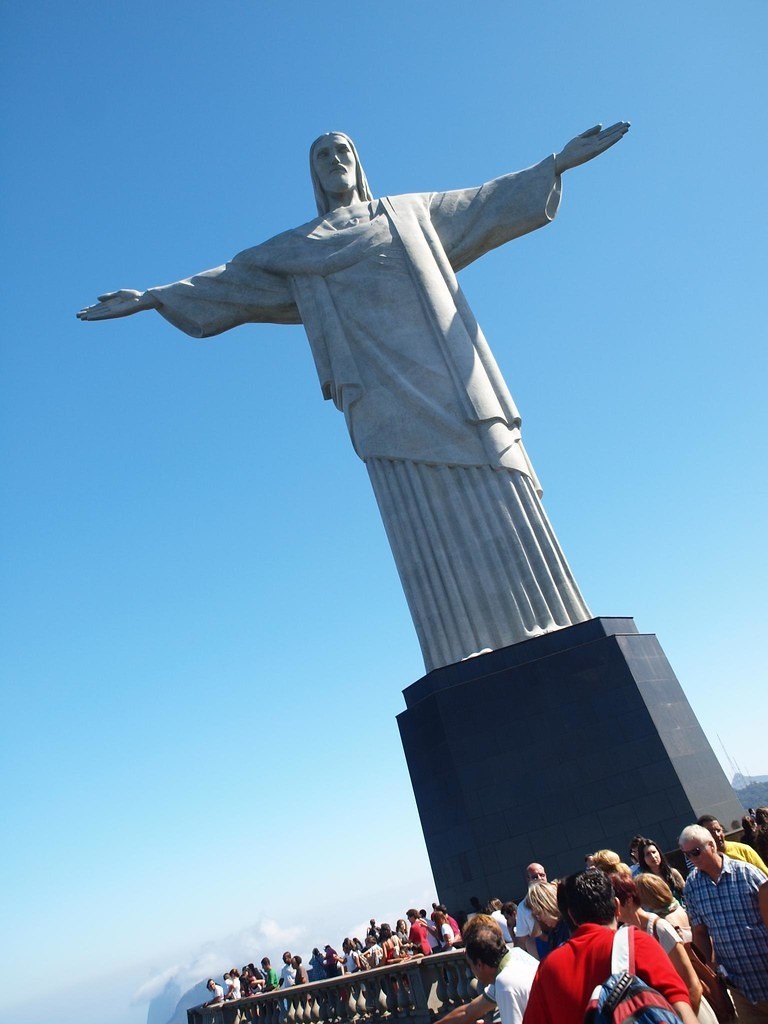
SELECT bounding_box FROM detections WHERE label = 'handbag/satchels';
[352,952,370,970]
[394,935,400,956]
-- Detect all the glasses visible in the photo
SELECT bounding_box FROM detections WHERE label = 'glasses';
[631,851,639,856]
[683,841,707,859]
[534,909,542,919]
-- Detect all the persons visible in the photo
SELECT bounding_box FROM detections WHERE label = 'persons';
[675,824,768,1024]
[516,867,699,1024]
[77,120,631,677]
[203,806,768,1024]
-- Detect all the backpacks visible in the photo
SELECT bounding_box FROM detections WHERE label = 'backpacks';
[585,926,684,1024]
